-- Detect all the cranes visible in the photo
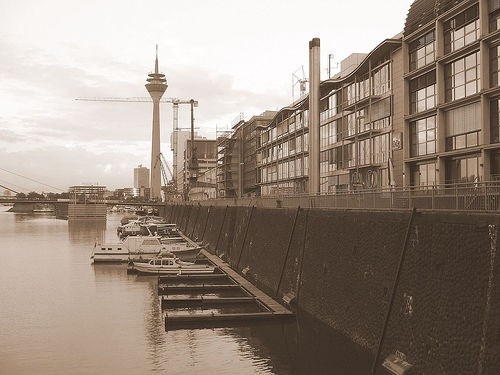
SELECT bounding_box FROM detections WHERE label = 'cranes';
[74,98,200,190]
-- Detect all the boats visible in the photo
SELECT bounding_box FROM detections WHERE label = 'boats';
[90,234,203,262]
[117,220,177,238]
[131,247,215,274]
[127,215,165,224]
